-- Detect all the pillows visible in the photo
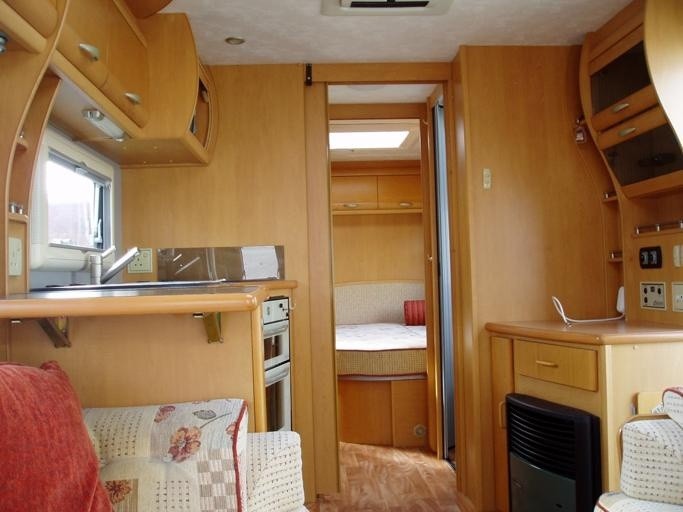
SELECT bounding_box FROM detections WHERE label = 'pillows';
[403,299,426,327]
[619,418,683,505]
[79,397,250,511]
[593,490,683,512]
[245,430,307,511]
[661,387,683,429]
[1,359,115,511]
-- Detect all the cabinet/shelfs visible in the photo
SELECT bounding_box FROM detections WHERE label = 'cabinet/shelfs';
[485,320,683,511]
[376,166,424,213]
[54,0,154,130]
[330,166,378,214]
[2,45,61,224]
[576,1,683,199]
[112,10,221,168]
[338,377,427,450]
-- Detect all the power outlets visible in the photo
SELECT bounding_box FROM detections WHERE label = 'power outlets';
[127,247,153,274]
[7,236,22,277]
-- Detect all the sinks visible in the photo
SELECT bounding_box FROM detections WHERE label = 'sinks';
[30,278,226,291]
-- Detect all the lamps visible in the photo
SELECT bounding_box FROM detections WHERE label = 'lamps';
[82,111,130,144]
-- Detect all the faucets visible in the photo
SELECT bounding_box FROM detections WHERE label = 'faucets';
[91,244,139,284]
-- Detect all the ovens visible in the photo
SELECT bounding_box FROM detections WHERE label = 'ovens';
[262,320,291,432]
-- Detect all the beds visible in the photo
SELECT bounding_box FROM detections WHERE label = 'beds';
[333,278,428,377]
